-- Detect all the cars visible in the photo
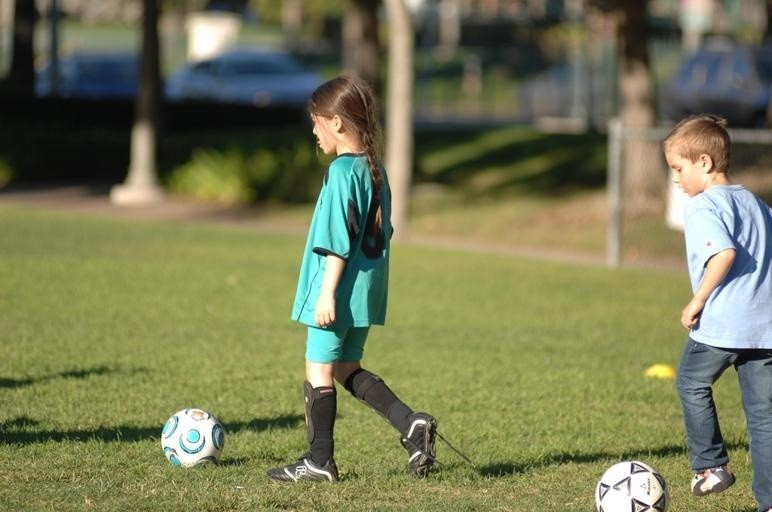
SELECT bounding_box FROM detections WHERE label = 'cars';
[517,45,772,124]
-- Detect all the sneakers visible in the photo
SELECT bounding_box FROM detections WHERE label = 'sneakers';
[689,466,737,496]
[400,410,437,478]
[265,450,338,485]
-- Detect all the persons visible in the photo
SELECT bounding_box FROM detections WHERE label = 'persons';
[662,113,771,511]
[261,73,437,483]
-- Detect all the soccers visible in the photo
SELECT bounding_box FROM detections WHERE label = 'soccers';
[595,460,670,512]
[161,408,226,469]
